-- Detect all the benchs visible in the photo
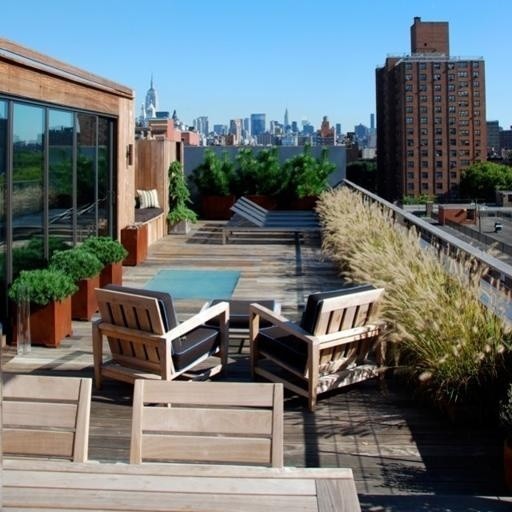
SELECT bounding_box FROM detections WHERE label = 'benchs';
[135,196,164,248]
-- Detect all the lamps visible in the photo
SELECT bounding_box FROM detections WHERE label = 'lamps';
[126,143,134,166]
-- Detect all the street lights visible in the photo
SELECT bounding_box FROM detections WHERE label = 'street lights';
[469,202,489,233]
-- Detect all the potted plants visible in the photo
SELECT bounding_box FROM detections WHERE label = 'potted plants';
[193,141,333,218]
[72,233,128,286]
[10,267,76,349]
[165,159,198,234]
[49,249,106,322]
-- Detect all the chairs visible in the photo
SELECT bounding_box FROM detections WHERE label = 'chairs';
[251,279,391,411]
[127,376,286,470]
[1,368,97,462]
[91,280,232,408]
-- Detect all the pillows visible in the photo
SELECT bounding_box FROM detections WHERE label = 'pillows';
[135,185,161,210]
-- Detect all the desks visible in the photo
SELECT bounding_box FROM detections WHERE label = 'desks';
[0,453,363,512]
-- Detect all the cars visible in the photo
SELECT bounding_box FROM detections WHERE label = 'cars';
[493,220,504,231]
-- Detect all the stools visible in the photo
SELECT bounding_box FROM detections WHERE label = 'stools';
[198,296,283,357]
